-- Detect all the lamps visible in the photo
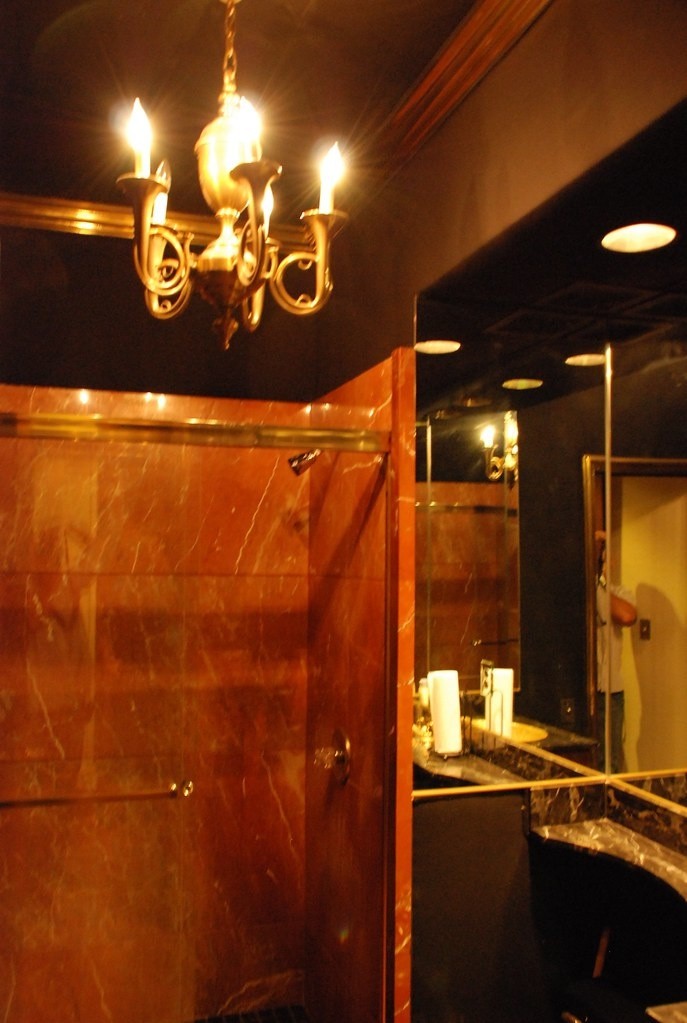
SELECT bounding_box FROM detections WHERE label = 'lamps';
[116,1,349,351]
[479,411,519,491]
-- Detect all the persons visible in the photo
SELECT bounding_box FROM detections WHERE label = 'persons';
[598,532,638,775]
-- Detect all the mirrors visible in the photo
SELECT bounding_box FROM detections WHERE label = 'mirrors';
[412,305,687,817]
